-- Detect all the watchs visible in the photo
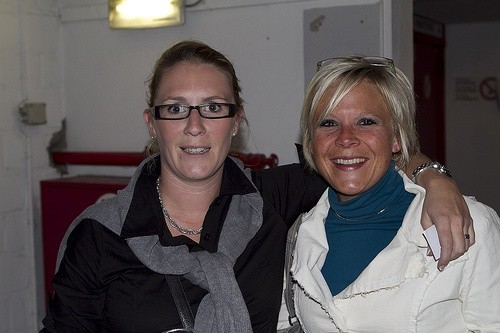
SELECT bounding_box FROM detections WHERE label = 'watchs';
[411,161,454,184]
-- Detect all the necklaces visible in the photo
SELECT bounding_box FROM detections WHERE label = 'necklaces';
[155,176,204,235]
[330,184,405,221]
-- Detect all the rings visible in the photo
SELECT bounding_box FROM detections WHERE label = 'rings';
[463,233,470,239]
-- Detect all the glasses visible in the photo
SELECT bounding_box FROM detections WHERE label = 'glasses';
[317,55,397,78]
[145,101,238,120]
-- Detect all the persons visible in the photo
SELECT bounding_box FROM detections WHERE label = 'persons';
[35,39,476,333]
[275,55,500,333]
